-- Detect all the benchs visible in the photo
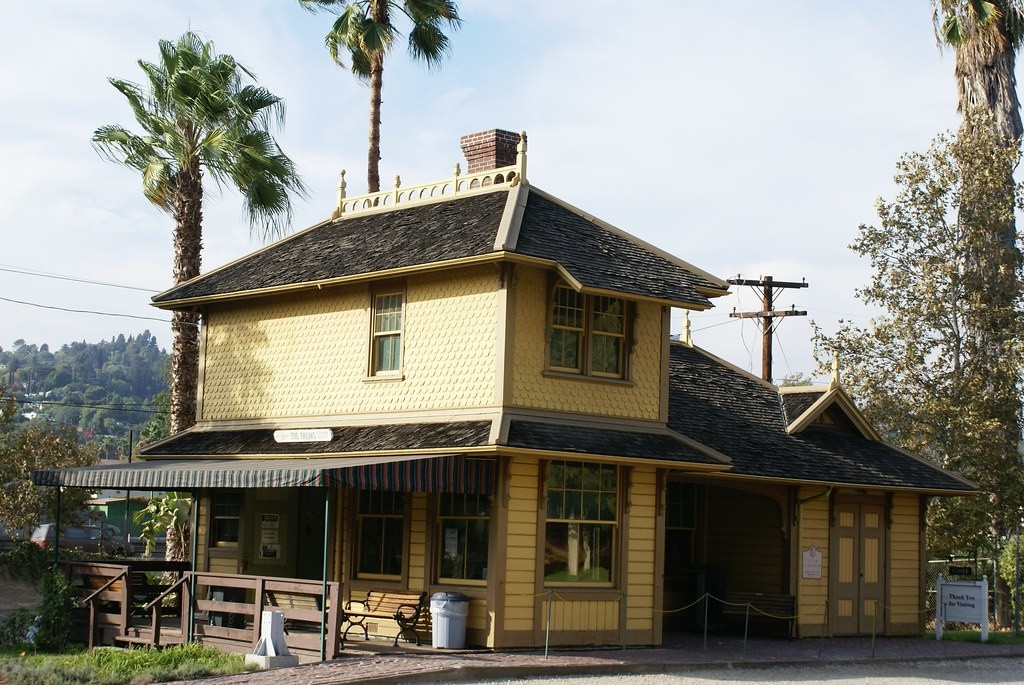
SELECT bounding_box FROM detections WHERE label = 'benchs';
[83,574,153,626]
[344,591,428,647]
[716,590,796,642]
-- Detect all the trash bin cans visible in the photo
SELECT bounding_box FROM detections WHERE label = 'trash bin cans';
[428,591,470,649]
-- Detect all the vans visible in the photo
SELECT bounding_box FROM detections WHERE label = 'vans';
[31,523,135,557]
